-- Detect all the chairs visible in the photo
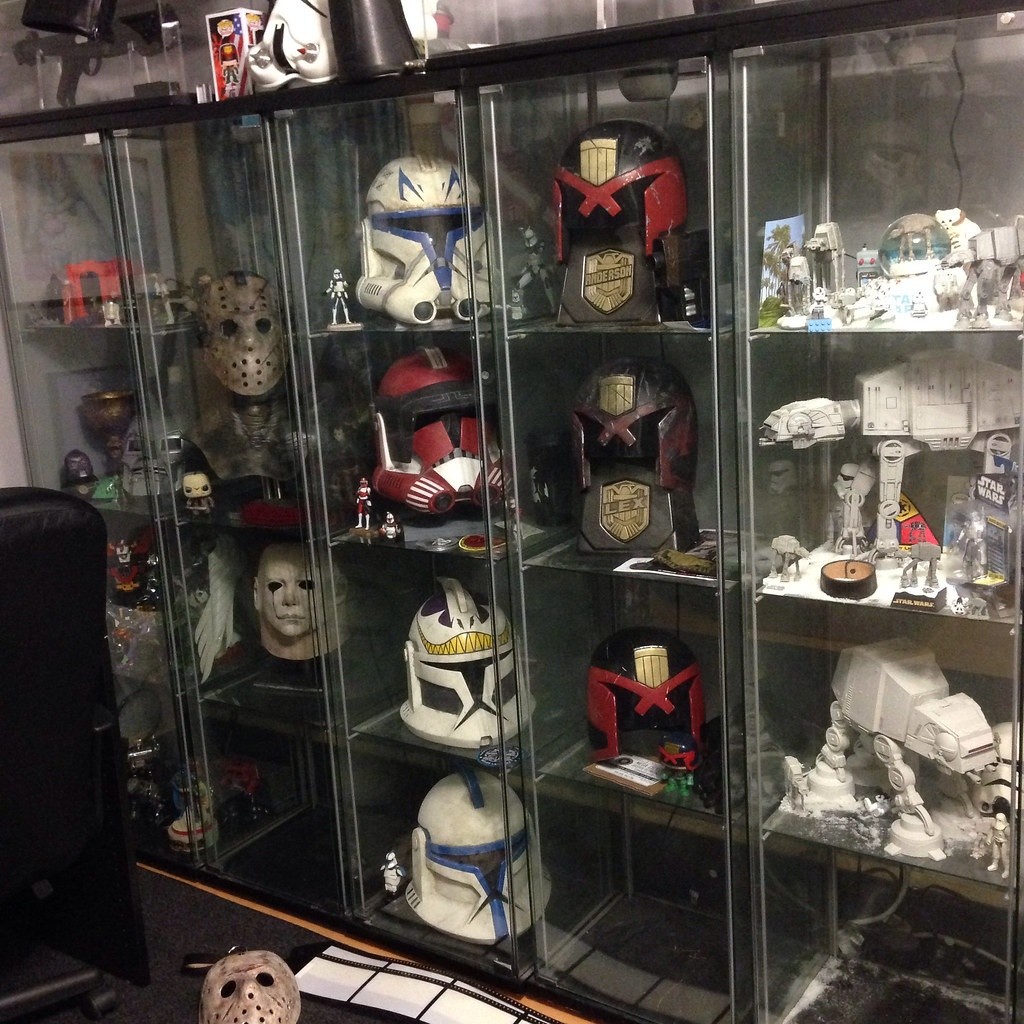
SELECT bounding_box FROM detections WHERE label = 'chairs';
[0,488,149,988]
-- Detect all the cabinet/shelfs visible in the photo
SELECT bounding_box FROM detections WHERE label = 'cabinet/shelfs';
[0,1,1022,1023]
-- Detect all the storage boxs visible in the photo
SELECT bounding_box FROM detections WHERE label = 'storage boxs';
[205,7,265,102]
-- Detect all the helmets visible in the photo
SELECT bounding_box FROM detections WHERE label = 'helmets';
[352,154,497,327]
[548,117,688,266]
[393,576,536,748]
[570,354,698,497]
[369,346,513,514]
[402,763,552,947]
[582,626,708,764]
